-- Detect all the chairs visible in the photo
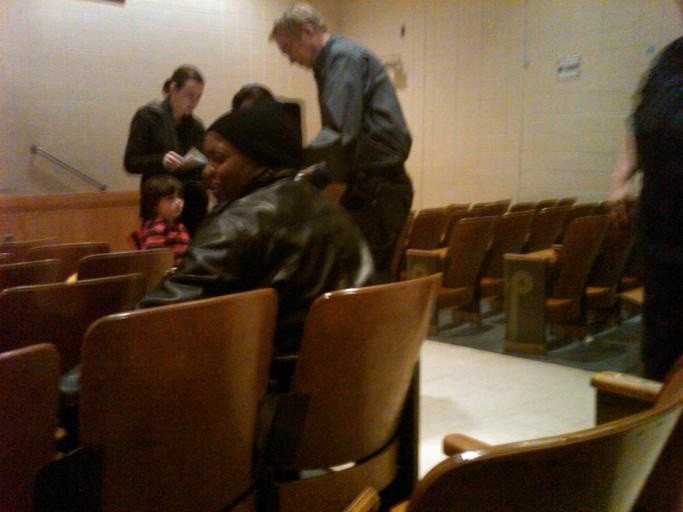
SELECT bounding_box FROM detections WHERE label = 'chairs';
[405,193,642,352]
[398,369,683,512]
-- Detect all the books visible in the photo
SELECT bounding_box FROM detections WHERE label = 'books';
[180,147,207,166]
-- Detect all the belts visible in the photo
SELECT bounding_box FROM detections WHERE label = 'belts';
[348,164,403,180]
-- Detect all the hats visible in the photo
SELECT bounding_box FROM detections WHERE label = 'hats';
[202,103,302,168]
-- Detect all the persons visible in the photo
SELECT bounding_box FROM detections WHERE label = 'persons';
[59,99,376,450]
[232,86,275,109]
[124,64,209,233]
[270,1,414,275]
[126,176,193,267]
[624,37,679,512]
